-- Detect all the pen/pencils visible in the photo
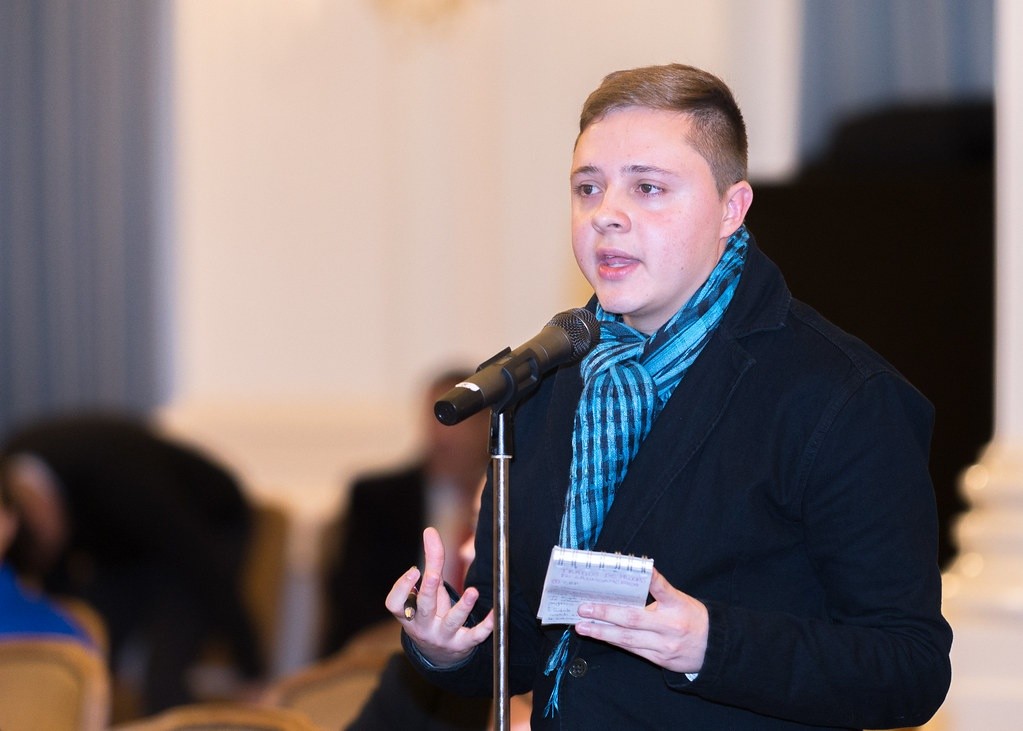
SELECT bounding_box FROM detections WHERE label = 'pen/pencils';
[403,575,422,621]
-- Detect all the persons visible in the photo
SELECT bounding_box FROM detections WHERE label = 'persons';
[315,369,494,661]
[383,63,953,731]
[0,406,274,723]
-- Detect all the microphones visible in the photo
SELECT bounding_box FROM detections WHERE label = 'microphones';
[434,307,600,426]
